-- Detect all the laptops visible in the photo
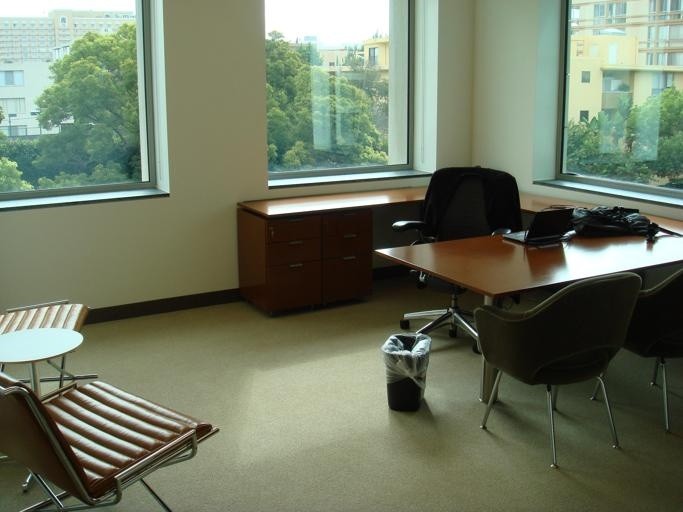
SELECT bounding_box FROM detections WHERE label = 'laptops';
[503,208,574,244]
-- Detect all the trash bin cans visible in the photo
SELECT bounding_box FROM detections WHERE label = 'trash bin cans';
[381,332,432,412]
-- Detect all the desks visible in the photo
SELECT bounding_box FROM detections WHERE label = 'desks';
[236,187,682,401]
[0,327,85,493]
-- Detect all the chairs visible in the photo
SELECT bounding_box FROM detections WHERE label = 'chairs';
[0,299,99,393]
[1,371,219,511]
[586,271,682,436]
[475,272,641,468]
[390,166,522,355]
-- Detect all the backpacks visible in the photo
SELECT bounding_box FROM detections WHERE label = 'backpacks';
[574,205,663,242]
